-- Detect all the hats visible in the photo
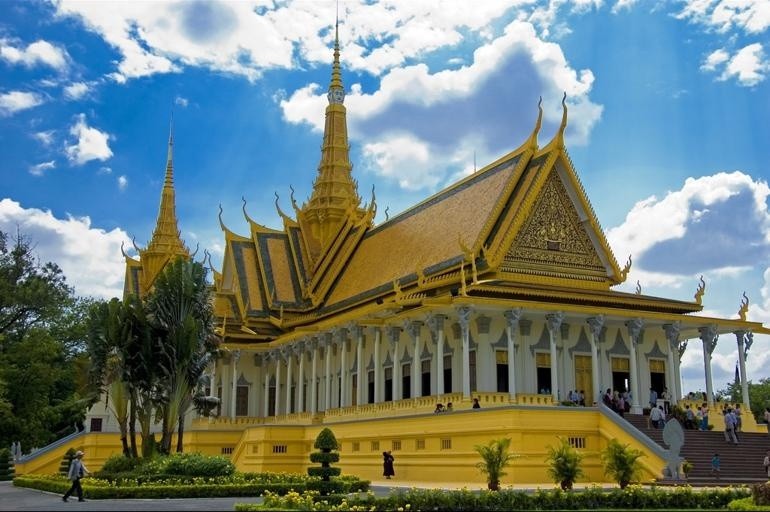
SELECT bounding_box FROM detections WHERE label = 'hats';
[75,450,85,456]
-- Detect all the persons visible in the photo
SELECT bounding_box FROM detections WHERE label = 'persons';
[767,409,770,435]
[763,450,770,477]
[657,405,667,429]
[578,390,585,405]
[472,398,480,408]
[720,402,742,444]
[648,403,661,429]
[382,450,395,478]
[709,453,721,481]
[661,386,671,414]
[573,389,578,404]
[61,450,93,502]
[696,407,703,431]
[446,402,454,411]
[763,408,769,424]
[568,390,572,401]
[601,387,634,418]
[683,404,694,429]
[701,402,710,431]
[647,387,657,407]
[433,403,446,413]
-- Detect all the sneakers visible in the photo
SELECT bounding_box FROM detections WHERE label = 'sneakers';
[77,498,86,502]
[62,496,70,502]
